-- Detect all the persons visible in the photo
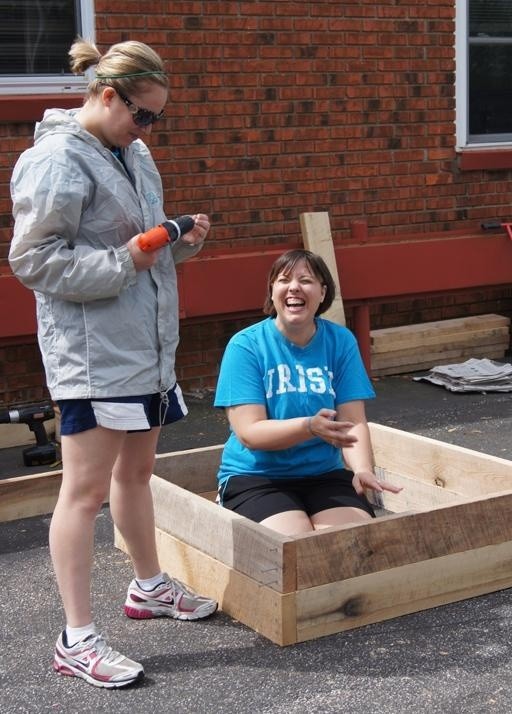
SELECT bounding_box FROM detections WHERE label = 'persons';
[210,248,405,537]
[5,34,219,692]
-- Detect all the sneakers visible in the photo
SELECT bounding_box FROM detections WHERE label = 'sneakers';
[53,630,144,688]
[124,577,218,620]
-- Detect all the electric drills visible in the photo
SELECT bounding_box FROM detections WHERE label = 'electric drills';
[136,215,195,252]
[0,405,57,467]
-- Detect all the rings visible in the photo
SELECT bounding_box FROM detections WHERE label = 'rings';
[376,479,380,484]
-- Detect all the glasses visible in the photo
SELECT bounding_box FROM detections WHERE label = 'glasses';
[117,89,165,127]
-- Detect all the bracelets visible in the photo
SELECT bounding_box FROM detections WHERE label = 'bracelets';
[307,415,318,437]
